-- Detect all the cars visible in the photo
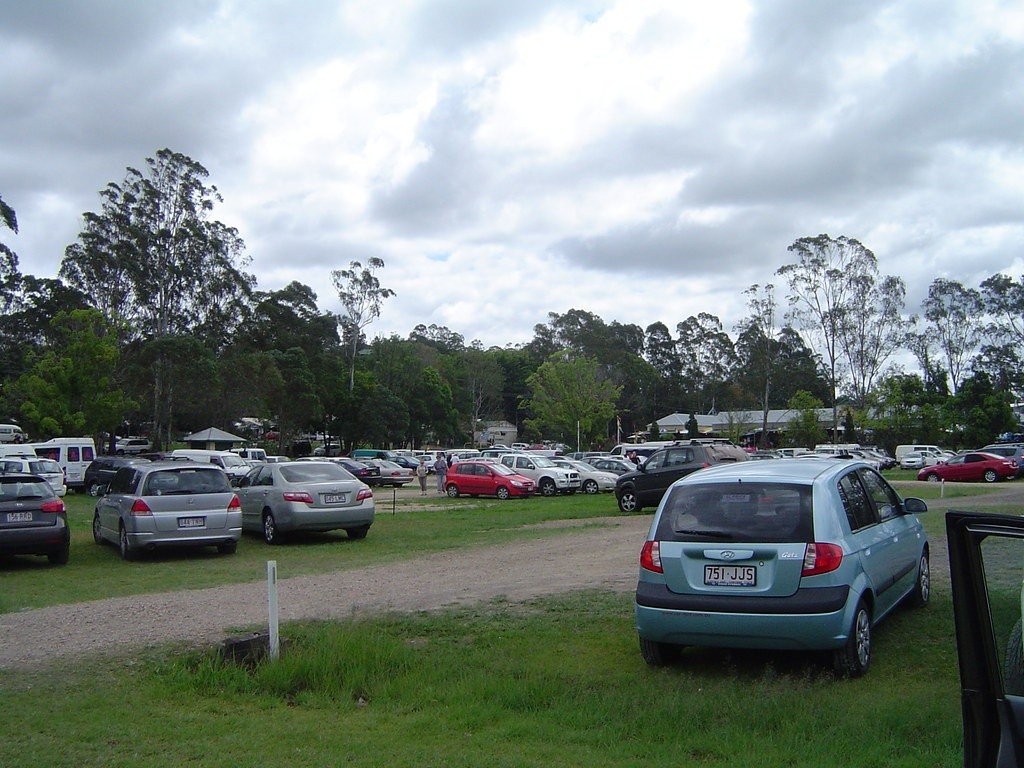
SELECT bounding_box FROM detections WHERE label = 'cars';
[751,442,896,470]
[633,454,932,679]
[91,460,243,559]
[443,461,536,500]
[896,442,1024,484]
[918,452,1021,483]
[230,461,375,544]
[0,470,70,566]
[313,444,341,457]
[0,434,691,498]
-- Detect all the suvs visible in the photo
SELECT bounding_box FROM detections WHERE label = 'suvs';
[615,438,752,513]
[499,454,581,497]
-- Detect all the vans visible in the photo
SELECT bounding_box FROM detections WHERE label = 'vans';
[0,424,26,444]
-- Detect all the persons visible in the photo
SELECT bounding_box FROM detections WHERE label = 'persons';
[265,429,274,440]
[11,432,28,443]
[416,458,430,495]
[432,455,450,493]
[626,451,641,465]
[446,451,459,466]
